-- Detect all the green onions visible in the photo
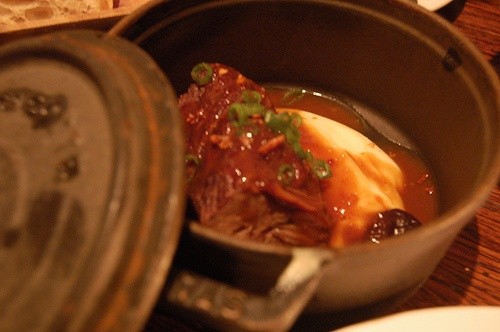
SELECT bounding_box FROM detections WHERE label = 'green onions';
[184,63,333,185]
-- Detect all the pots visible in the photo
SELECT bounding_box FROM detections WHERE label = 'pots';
[103,1,500,330]
